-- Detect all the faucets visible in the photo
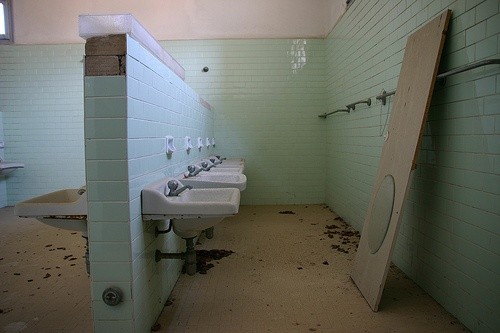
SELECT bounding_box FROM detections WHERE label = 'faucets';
[207,165,215,171]
[214,162,222,166]
[77,187,87,195]
[176,185,193,194]
[195,167,205,175]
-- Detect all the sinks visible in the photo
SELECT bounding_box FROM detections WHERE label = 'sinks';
[209,174,241,183]
[183,190,234,202]
[213,168,240,172]
[221,158,226,161]
[24,188,78,203]
